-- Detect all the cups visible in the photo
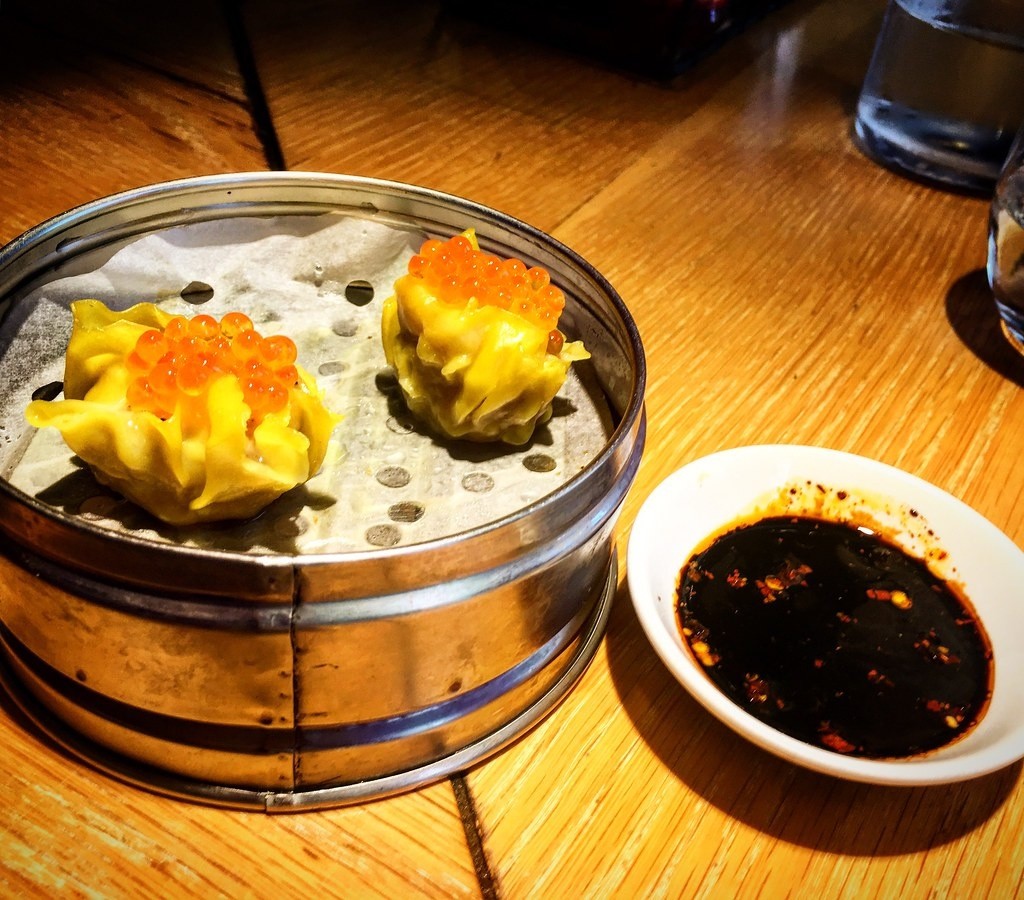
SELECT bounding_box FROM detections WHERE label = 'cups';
[853,0,1024,190]
[987,134,1024,355]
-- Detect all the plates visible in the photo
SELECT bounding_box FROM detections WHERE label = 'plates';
[629,444,1024,785]
